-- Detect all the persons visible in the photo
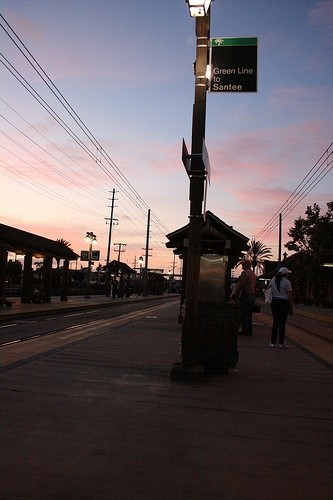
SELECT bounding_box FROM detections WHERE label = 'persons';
[268,267,293,347]
[111,276,119,300]
[236,261,256,336]
[231,281,236,299]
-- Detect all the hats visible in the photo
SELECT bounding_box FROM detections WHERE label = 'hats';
[241,260,251,267]
[279,267,291,275]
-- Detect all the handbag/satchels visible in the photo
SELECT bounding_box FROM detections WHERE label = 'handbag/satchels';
[252,302,261,313]
[262,287,272,304]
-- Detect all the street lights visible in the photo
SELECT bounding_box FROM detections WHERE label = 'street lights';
[169,0,211,382]
[137,256,146,296]
[84,231,98,300]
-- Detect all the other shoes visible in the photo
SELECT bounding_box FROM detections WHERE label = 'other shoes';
[270,341,277,348]
[238,330,252,336]
[279,343,289,348]
